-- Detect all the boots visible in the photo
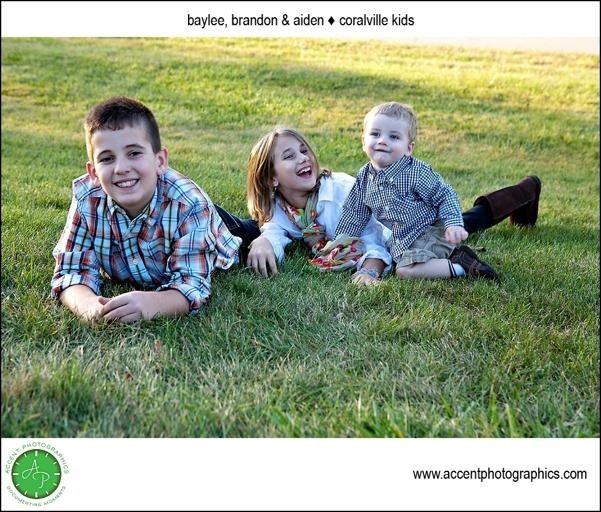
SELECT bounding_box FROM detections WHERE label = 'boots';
[473,175,542,226]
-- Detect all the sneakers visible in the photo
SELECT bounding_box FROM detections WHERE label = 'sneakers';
[448,245,498,280]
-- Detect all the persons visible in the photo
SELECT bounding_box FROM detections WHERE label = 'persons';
[48,96,254,325]
